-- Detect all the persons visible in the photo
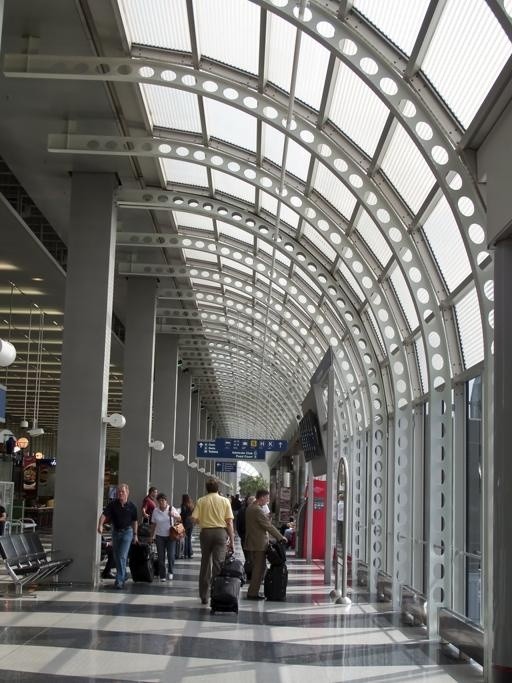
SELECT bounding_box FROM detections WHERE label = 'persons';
[98,479,296,604]
[0,506,8,563]
[337,494,344,544]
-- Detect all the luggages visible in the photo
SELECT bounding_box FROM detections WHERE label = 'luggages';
[129,559,154,582]
[210,576,242,615]
[264,565,288,602]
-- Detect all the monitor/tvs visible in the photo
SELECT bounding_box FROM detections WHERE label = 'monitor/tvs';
[298,409,323,463]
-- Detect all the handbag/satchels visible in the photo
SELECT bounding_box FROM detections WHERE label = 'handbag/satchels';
[128,542,151,560]
[266,542,286,566]
[170,523,186,542]
[137,520,156,537]
[221,560,248,587]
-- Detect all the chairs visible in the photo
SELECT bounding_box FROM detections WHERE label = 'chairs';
[1,532,74,596]
